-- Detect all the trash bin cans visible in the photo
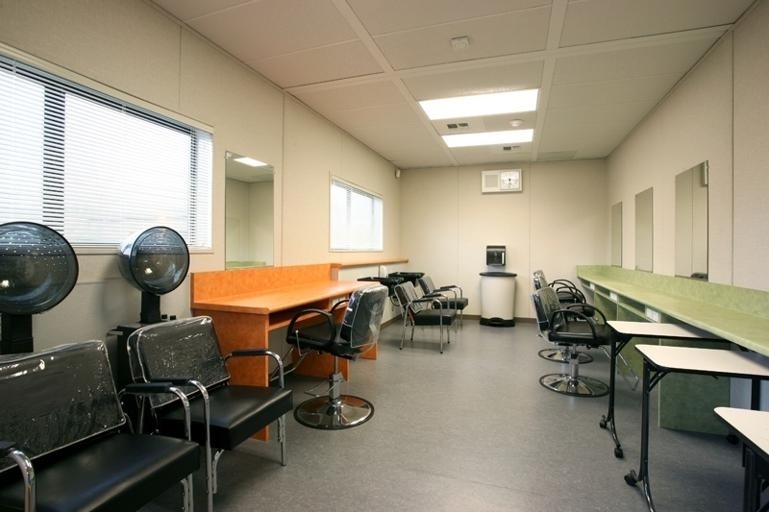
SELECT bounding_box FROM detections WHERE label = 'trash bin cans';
[480,272,517,327]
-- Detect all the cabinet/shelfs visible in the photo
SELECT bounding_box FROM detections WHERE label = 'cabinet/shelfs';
[330,260,409,326]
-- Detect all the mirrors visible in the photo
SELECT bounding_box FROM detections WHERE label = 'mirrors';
[610,161,708,281]
[225,151,275,270]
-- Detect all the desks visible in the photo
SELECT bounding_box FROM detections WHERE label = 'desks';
[623,344,768,511]
[192,280,380,442]
[714,406,767,509]
[599,320,761,468]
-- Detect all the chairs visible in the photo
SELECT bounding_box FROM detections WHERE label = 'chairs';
[0,340,201,510]
[287,286,389,430]
[127,316,292,509]
[532,271,610,397]
[394,274,470,353]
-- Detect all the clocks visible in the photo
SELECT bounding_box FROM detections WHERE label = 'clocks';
[500,168,523,193]
